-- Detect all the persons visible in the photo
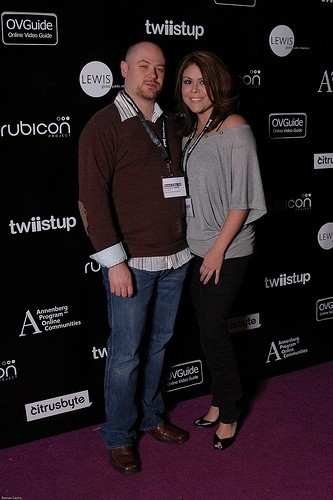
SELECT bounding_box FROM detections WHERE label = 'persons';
[168,52,268,450]
[77,40,194,476]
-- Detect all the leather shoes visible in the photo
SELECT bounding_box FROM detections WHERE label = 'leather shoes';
[109,445,139,475]
[147,422,188,443]
[194,413,221,428]
[214,419,238,450]
